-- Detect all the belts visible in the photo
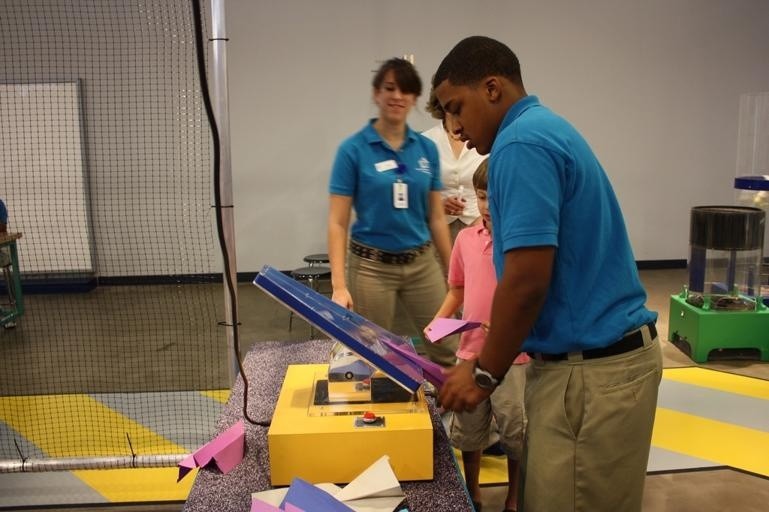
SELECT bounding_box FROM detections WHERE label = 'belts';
[526,318,658,362]
[348,240,430,266]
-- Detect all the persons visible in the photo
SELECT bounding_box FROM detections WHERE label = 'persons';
[418,76,492,319]
[424,156,532,512]
[433,36,662,511]
[327,56,506,456]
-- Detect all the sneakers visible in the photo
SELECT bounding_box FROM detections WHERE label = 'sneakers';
[471,430,518,512]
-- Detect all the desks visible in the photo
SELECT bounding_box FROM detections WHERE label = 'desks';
[185,333,472,511]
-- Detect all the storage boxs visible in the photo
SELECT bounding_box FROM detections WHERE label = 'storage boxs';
[265,363,435,486]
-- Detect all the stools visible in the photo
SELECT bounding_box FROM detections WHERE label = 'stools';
[285,253,331,340]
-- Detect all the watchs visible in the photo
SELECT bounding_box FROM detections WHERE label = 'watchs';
[471,359,501,390]
